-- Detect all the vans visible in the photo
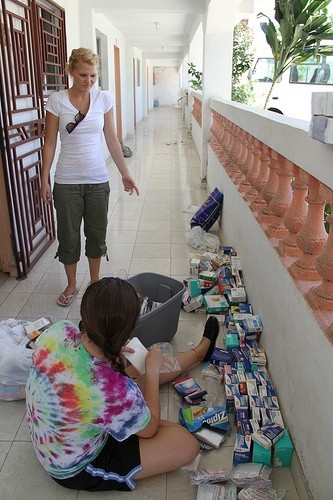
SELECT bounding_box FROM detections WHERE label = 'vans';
[243,53,333,117]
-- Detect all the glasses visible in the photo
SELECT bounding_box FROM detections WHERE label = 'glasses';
[65,110,85,134]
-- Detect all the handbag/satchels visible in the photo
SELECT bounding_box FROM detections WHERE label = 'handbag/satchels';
[0,318,49,401]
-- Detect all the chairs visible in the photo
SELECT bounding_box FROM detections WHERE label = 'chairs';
[309,64,330,83]
[292,67,298,82]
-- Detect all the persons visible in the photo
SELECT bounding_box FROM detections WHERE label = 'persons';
[41,48,140,306]
[26,276,219,490]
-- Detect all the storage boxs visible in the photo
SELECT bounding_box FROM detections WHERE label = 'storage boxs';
[182,246,293,468]
[124,273,186,347]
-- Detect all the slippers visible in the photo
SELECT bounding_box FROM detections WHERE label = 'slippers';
[57,288,79,306]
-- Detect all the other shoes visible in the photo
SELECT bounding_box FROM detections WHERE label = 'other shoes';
[201,316,219,362]
